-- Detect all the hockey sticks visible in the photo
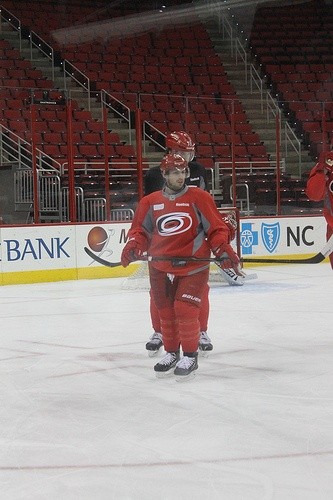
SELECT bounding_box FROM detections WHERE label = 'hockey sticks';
[149,235,333,264]
[84,246,122,269]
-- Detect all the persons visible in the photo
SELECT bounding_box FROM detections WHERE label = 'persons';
[306,130,333,270]
[121,131,247,376]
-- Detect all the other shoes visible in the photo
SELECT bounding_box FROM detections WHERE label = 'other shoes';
[216,268,245,286]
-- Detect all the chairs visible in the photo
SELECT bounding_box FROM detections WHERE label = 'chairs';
[0,0,333,221]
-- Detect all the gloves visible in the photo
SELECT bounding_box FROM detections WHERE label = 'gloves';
[121,237,145,268]
[213,242,239,268]
[314,151,333,173]
[220,211,237,241]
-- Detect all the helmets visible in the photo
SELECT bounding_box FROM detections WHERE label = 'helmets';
[160,154,187,174]
[166,131,195,151]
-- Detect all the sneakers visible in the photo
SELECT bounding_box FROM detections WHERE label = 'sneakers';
[174,352,198,383]
[145,333,163,358]
[198,331,213,358]
[154,349,180,378]
